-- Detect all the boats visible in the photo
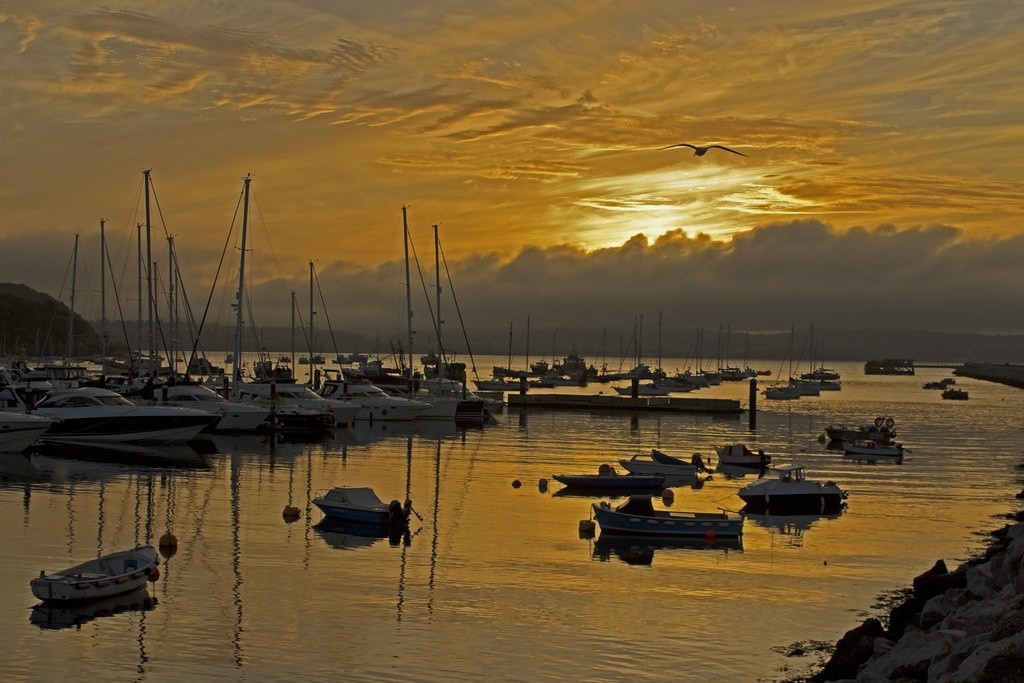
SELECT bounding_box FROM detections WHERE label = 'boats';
[617,442,772,481]
[737,461,848,515]
[592,496,743,548]
[923,378,956,390]
[312,487,410,538]
[941,388,968,400]
[552,465,665,496]
[30,544,159,601]
[825,417,903,455]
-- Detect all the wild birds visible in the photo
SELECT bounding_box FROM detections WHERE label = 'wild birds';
[656,143,748,157]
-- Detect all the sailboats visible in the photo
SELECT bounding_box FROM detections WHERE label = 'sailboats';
[0,169,841,453]
